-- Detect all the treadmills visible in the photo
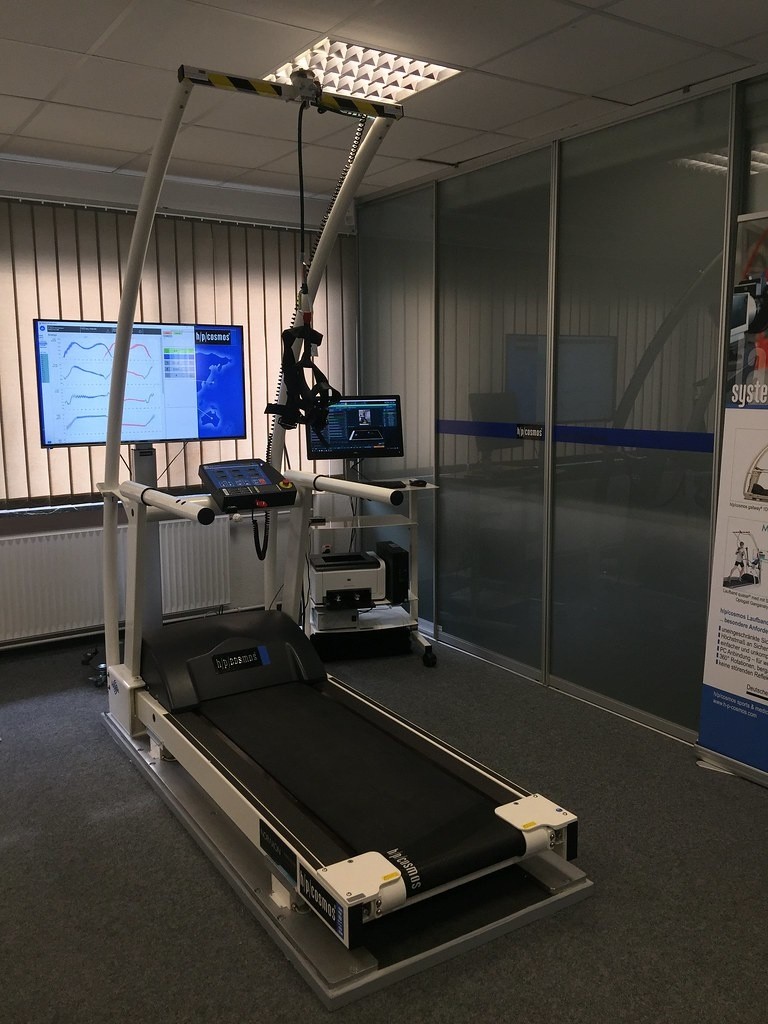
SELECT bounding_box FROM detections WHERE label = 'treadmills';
[101,61,597,1008]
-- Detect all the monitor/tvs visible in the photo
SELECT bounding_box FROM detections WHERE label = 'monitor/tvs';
[730,292,749,344]
[468,393,524,450]
[502,333,619,422]
[32,319,247,449]
[734,283,756,299]
[306,395,404,460]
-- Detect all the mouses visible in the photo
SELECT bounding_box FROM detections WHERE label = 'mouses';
[410,480,427,487]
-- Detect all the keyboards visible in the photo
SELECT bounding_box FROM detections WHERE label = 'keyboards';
[358,481,407,488]
